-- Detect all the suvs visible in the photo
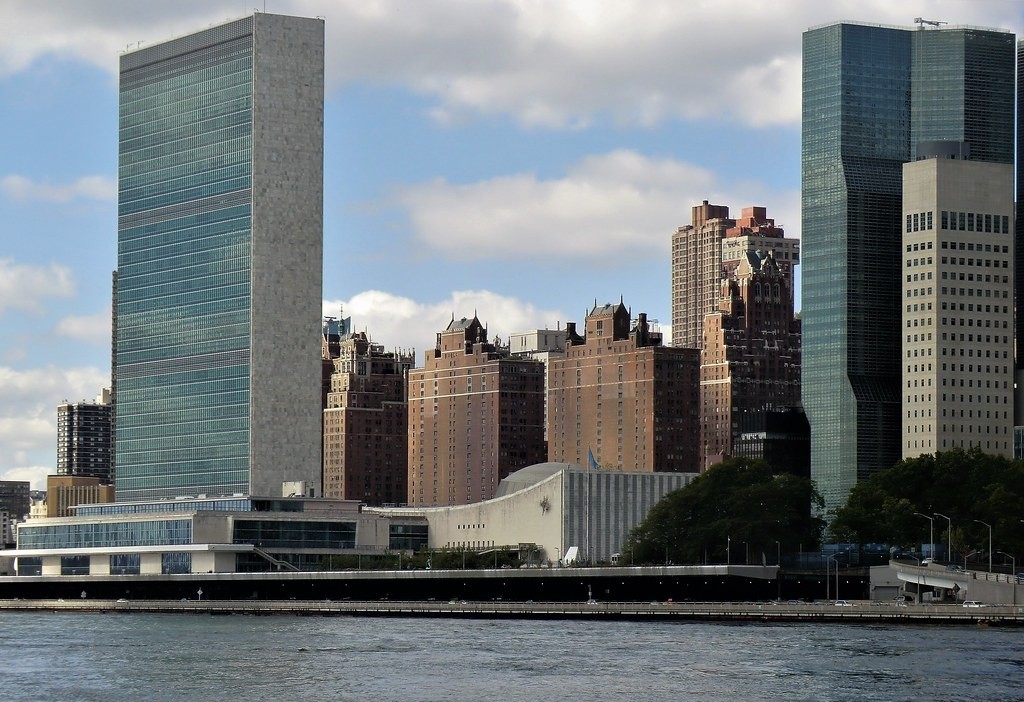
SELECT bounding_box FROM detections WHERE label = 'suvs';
[962,600,986,609]
[963,601,985,608]
[836,600,856,607]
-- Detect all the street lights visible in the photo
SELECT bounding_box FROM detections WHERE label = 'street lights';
[974,520,992,573]
[914,513,934,562]
[965,552,981,570]
[827,552,845,600]
[998,552,1016,604]
[743,542,748,564]
[822,555,838,600]
[554,547,559,568]
[934,513,951,561]
[776,541,780,564]
[398,552,401,569]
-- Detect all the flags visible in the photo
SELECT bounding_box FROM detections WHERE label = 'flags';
[589,450,601,470]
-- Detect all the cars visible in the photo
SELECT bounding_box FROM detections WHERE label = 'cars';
[117,599,129,603]
[788,600,806,605]
[871,601,888,606]
[922,557,934,566]
